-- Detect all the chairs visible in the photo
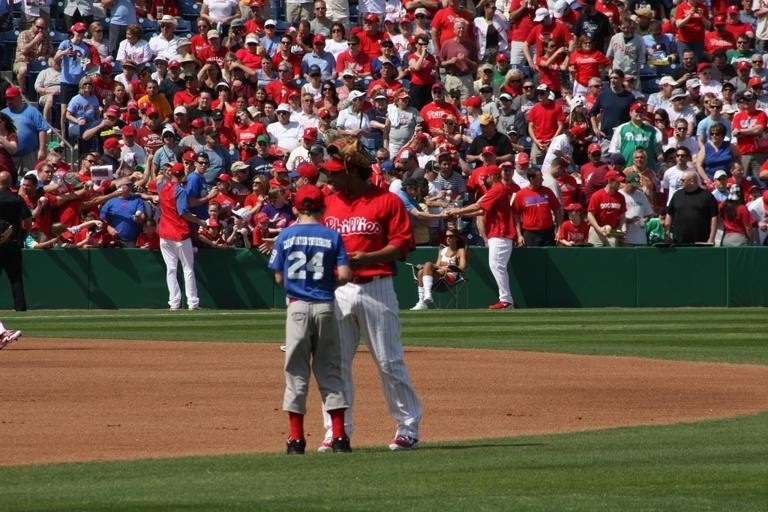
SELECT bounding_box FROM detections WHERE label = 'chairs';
[413,242,471,310]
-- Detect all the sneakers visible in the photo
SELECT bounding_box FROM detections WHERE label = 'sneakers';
[332,435,352,453]
[318,436,332,453]
[285,433,308,456]
[489,301,515,312]
[0,329,23,349]
[388,436,418,451]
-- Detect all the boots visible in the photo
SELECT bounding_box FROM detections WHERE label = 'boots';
[423,274,436,305]
[411,285,429,311]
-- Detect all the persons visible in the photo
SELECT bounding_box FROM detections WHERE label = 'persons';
[316,135,424,452]
[267,183,353,454]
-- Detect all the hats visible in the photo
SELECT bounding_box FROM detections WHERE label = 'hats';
[0,0,768,248]
